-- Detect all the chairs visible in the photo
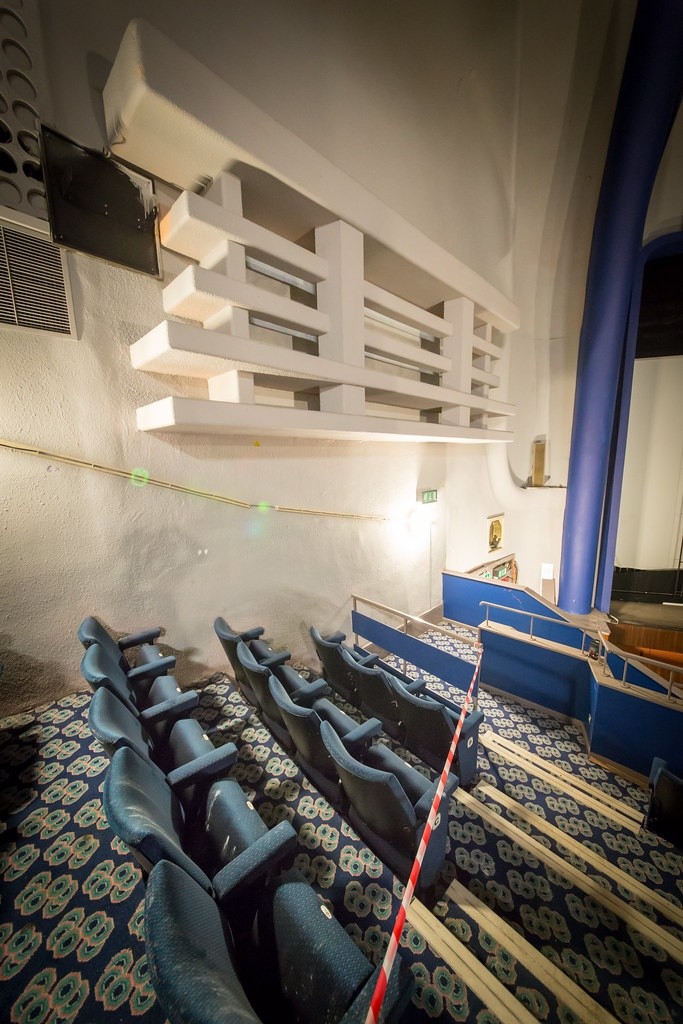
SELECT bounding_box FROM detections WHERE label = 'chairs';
[214,617,291,716]
[102,742,298,902]
[386,674,484,792]
[80,644,199,749]
[310,627,379,710]
[645,757,683,848]
[87,688,239,807]
[236,641,328,759]
[268,675,382,813]
[320,718,459,895]
[341,649,426,746]
[144,860,416,1024]
[76,616,175,701]
